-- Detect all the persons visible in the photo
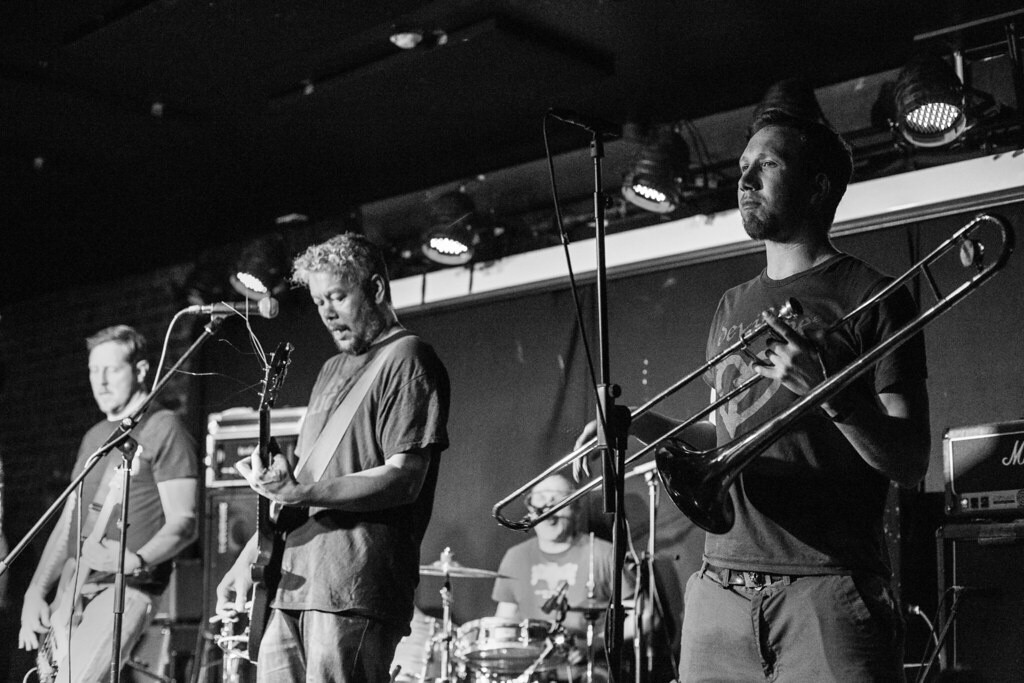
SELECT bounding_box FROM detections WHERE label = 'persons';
[571,80,931,683]
[16,323,205,683]
[489,469,661,683]
[214,231,451,682]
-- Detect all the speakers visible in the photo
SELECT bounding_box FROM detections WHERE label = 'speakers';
[932,522,1024,683]
[200,487,258,627]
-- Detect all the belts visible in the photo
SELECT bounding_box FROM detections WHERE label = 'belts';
[704,562,797,589]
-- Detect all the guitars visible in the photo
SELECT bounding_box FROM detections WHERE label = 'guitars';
[32,443,145,683]
[246,340,298,661]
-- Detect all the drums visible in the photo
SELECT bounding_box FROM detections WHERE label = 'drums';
[455,615,571,672]
[465,658,552,683]
[392,611,460,683]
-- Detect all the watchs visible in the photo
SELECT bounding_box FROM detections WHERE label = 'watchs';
[133,553,149,580]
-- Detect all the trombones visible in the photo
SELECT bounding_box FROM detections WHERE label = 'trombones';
[491,213,1013,543]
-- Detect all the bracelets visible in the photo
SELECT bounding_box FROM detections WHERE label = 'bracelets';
[819,402,852,423]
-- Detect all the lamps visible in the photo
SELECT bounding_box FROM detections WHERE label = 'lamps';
[296,78,314,95]
[388,23,449,50]
[620,112,718,216]
[417,193,508,266]
[227,231,311,301]
[887,48,1000,154]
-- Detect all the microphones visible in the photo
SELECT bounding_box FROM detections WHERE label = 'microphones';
[186,296,279,319]
[950,580,997,599]
[543,581,568,615]
[523,503,559,527]
[548,106,623,139]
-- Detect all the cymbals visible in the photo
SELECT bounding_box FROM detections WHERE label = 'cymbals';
[419,563,513,579]
[566,600,634,614]
